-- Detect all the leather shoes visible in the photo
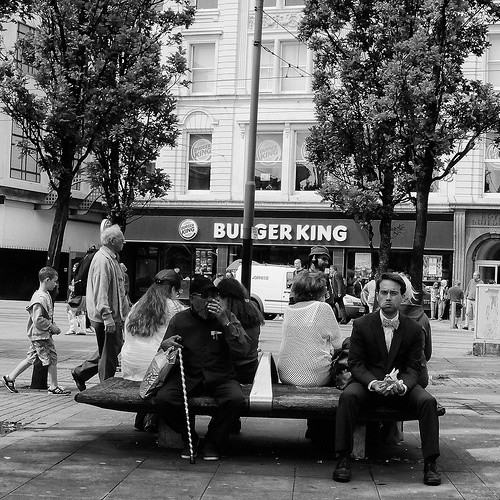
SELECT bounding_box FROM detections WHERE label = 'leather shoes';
[424,469,442,485]
[333,461,352,482]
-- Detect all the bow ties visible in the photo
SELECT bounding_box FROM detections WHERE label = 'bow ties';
[382,318,400,330]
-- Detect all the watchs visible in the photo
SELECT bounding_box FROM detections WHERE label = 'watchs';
[223,322,230,328]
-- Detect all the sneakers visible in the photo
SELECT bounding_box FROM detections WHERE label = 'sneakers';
[48,385,71,396]
[2,375,18,393]
[86,327,93,333]
[202,437,219,461]
[76,329,86,335]
[180,432,199,459]
[65,329,75,335]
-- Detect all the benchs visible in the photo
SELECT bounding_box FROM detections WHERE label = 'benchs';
[72,375,446,447]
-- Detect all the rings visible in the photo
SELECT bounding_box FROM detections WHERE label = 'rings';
[218,306,220,309]
[217,309,220,313]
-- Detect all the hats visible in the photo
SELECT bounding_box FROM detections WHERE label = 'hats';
[308,246,331,259]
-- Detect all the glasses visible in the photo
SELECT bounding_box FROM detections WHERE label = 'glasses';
[316,256,331,263]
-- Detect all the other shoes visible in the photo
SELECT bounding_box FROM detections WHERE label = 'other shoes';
[431,317,474,331]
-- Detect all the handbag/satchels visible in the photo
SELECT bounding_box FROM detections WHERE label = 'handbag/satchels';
[139,346,179,400]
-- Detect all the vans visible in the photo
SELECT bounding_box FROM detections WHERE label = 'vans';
[237,265,365,323]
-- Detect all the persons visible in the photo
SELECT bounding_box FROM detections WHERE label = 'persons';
[154,276,254,461]
[216,277,265,435]
[352,275,377,314]
[120,269,188,434]
[289,244,333,307]
[64,263,88,335]
[293,259,306,278]
[70,224,127,393]
[397,274,432,389]
[332,272,442,486]
[328,264,350,325]
[429,270,496,331]
[276,268,343,443]
[1,267,72,396]
